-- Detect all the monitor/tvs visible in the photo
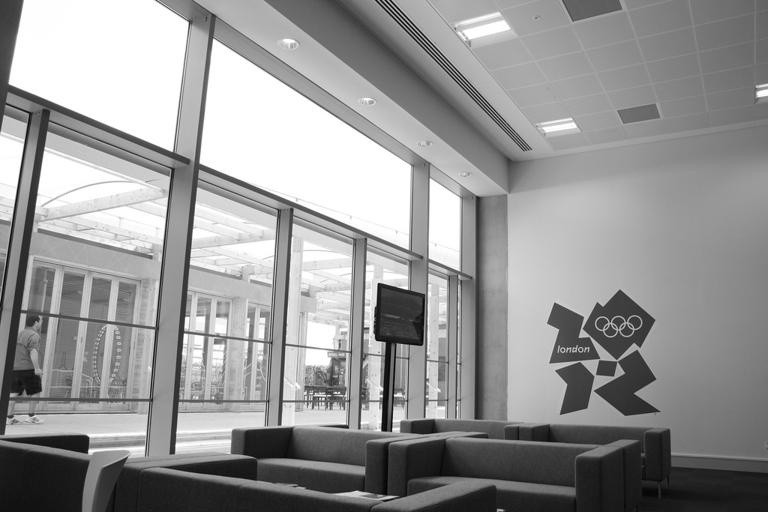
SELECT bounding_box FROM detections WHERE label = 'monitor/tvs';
[375,282,426,346]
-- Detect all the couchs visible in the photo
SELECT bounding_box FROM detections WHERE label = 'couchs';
[0,420,670,512]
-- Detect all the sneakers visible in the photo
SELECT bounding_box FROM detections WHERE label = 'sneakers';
[26,416,44,423]
[6,417,21,425]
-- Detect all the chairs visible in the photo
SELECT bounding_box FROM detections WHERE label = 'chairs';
[302,375,408,410]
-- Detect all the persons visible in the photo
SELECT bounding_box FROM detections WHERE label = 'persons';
[5,309,46,425]
